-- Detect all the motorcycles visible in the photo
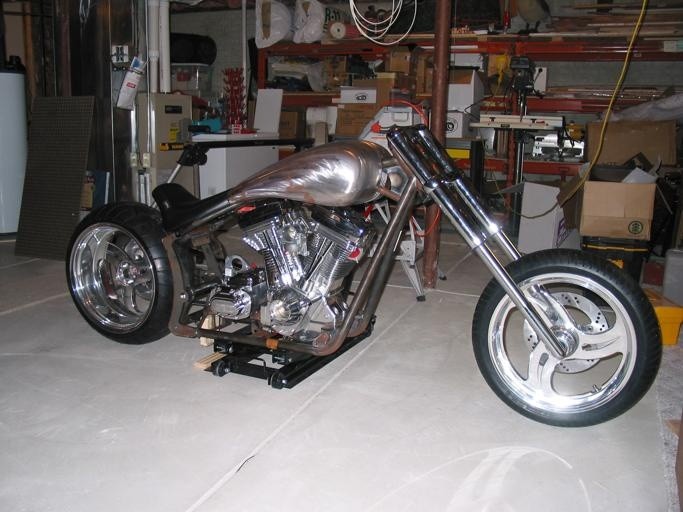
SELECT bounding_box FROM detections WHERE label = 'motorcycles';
[62,119,665,429]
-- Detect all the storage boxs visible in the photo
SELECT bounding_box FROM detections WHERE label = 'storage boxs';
[555,166,673,242]
